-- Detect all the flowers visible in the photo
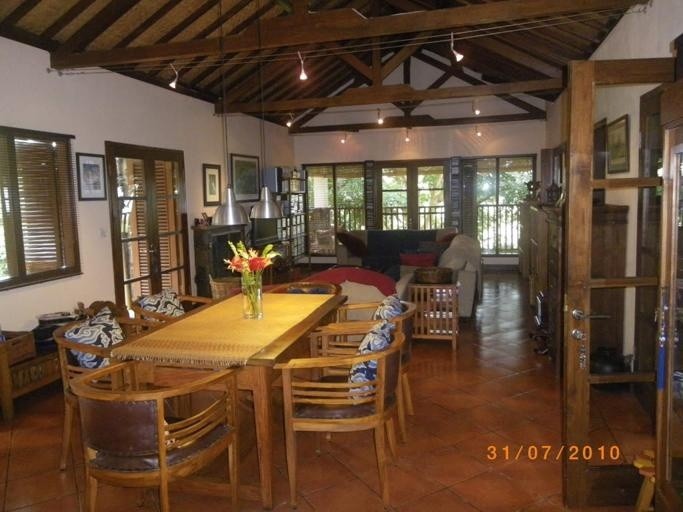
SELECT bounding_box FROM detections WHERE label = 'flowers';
[222,241,282,314]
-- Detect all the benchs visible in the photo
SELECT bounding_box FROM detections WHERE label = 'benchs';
[0,307,142,426]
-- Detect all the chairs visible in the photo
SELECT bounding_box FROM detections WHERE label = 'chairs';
[398,234,479,319]
[54,294,417,511]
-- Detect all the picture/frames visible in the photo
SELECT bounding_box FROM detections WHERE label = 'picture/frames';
[202,163,222,207]
[606,112,631,174]
[74,151,108,200]
[230,153,261,203]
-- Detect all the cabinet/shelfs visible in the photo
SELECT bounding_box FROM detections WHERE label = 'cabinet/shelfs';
[516,201,629,380]
[253,239,293,283]
[190,223,247,299]
[263,163,310,266]
[408,279,462,353]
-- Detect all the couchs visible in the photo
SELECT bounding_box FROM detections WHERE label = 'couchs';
[337,227,460,270]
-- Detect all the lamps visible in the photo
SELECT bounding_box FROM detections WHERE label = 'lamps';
[248,1,283,220]
[166,65,178,89]
[297,52,308,81]
[376,108,384,125]
[210,1,252,227]
[286,114,293,128]
[450,32,464,62]
[471,101,481,116]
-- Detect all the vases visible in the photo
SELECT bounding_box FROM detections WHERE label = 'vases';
[240,274,263,319]
